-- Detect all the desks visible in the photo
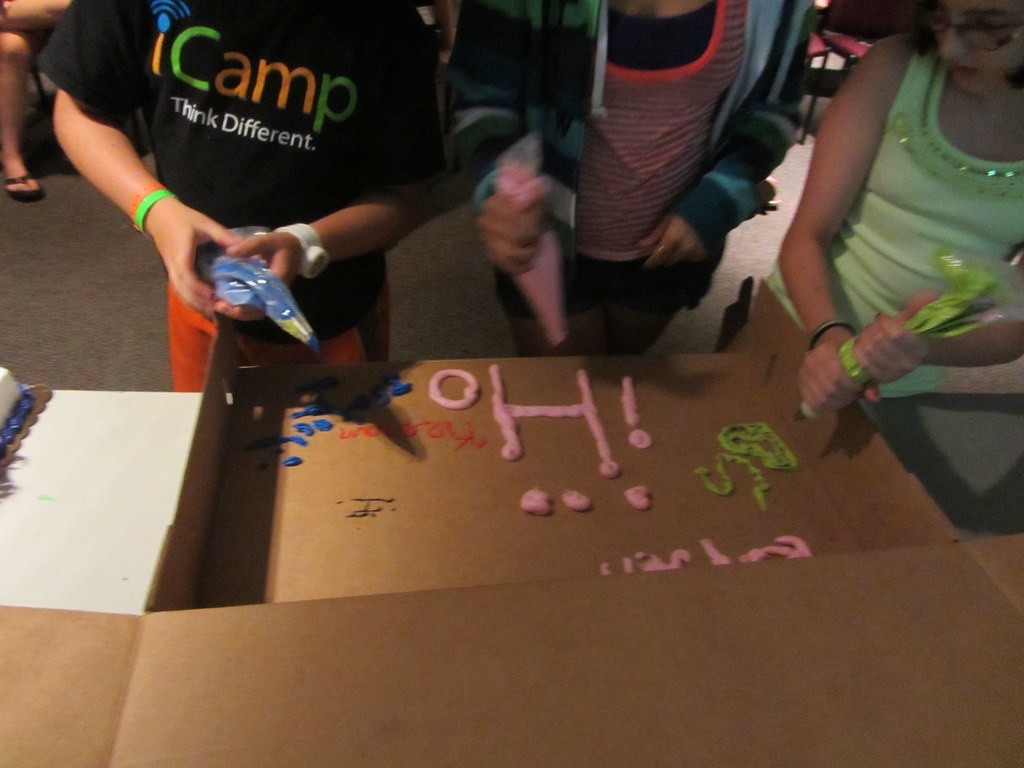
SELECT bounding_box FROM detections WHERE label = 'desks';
[1,380,206,615]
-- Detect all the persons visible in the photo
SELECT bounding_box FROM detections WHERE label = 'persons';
[442,0,816,357]
[725,0,1024,523]
[0,0,73,201]
[33,0,448,392]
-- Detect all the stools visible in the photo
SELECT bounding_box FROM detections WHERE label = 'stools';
[791,31,872,145]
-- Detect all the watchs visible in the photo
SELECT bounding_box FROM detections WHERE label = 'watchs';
[277,223,330,280]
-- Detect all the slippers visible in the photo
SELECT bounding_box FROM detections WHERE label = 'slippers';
[4,173,45,203]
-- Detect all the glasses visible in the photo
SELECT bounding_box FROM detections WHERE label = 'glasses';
[927,14,1024,53]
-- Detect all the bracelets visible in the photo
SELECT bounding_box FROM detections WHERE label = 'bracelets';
[129,184,178,237]
[810,318,857,350]
